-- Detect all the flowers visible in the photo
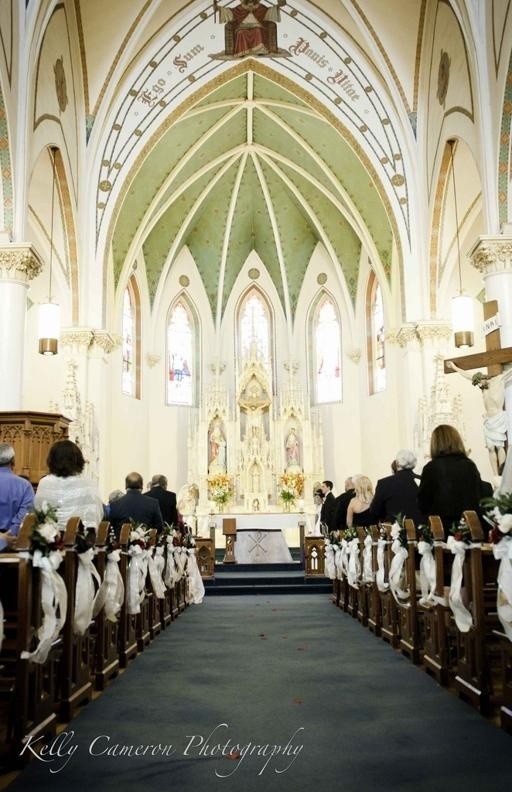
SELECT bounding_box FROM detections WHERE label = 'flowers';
[277,471,306,503]
[206,471,235,504]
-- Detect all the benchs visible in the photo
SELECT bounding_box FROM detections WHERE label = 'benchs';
[0,507,215,772]
[318,509,512,735]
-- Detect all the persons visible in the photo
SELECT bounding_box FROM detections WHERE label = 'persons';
[104,489,125,519]
[308,423,496,658]
[1,442,34,552]
[212,0,287,59]
[108,471,164,533]
[141,473,178,526]
[34,439,106,538]
[208,425,227,474]
[284,426,300,466]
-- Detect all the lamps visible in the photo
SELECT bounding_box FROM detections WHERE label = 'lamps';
[442,138,477,351]
[36,143,67,358]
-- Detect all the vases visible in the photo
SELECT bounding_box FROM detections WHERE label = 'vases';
[215,499,224,513]
[283,498,291,513]
